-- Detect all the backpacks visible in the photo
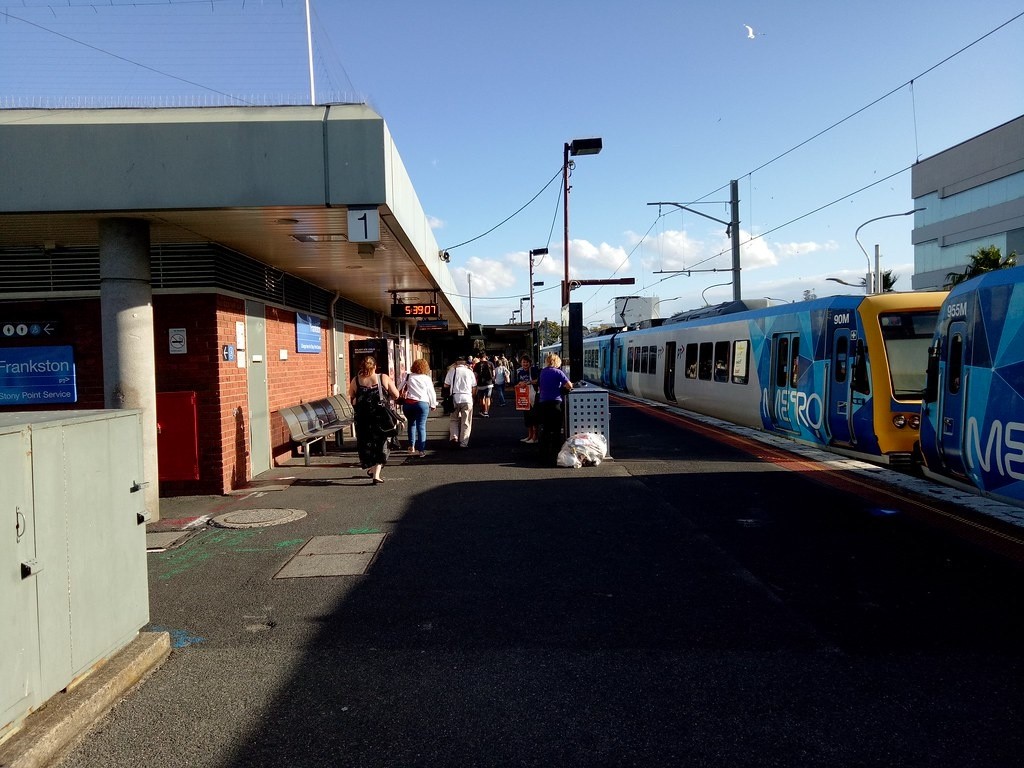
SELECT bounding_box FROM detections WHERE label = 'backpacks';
[478,361,493,386]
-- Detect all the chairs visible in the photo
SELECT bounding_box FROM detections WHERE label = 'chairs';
[277,393,355,466]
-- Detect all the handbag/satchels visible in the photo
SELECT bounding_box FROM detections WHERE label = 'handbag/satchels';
[441,396,455,414]
[514,385,531,412]
[397,374,409,404]
[376,402,406,435]
[503,366,510,383]
[529,367,541,407]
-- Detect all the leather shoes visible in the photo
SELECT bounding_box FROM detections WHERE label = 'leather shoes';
[373,478,384,486]
[367,470,373,477]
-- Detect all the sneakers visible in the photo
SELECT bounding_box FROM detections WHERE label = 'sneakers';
[409,446,414,452]
[520,438,538,444]
[419,452,426,457]
[478,411,489,418]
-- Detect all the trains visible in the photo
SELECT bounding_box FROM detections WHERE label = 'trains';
[542,287,954,470]
[916,266,1024,510]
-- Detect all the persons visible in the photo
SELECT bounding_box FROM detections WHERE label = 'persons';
[348,356,400,483]
[397,358,439,458]
[515,354,573,449]
[444,349,514,450]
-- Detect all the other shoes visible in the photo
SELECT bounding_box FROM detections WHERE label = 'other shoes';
[461,446,470,450]
[451,439,458,449]
[500,403,505,407]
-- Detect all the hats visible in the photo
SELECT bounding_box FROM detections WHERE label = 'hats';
[456,354,465,360]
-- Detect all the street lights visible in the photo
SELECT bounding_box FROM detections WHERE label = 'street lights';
[562,136,636,310]
[823,205,928,296]
[511,309,520,324]
[519,297,530,325]
[529,248,549,328]
